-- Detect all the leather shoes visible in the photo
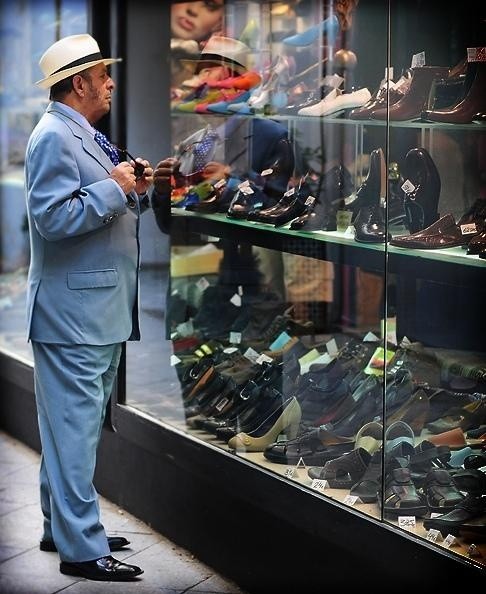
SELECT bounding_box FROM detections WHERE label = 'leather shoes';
[59,554,146,581]
[346,147,384,222]
[262,138,296,205]
[287,207,340,231]
[390,197,486,259]
[351,204,393,243]
[38,531,131,553]
[184,184,235,213]
[257,185,301,224]
[271,176,318,227]
[225,178,262,219]
[402,146,442,233]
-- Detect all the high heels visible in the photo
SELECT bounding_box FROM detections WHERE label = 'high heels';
[175,13,486,124]
[137,302,486,547]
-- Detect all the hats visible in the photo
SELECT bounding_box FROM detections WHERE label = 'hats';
[30,30,125,89]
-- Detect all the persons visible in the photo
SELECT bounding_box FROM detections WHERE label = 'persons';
[155,32,295,452]
[165,0,226,81]
[22,28,151,582]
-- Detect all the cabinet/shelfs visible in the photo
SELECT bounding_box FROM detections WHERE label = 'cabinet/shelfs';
[0,94,45,454]
[111,110,486,590]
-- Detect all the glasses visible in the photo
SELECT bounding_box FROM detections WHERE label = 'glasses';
[124,149,146,182]
[170,138,220,188]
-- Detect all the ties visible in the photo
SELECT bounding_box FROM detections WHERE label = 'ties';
[188,130,220,174]
[94,128,121,167]
